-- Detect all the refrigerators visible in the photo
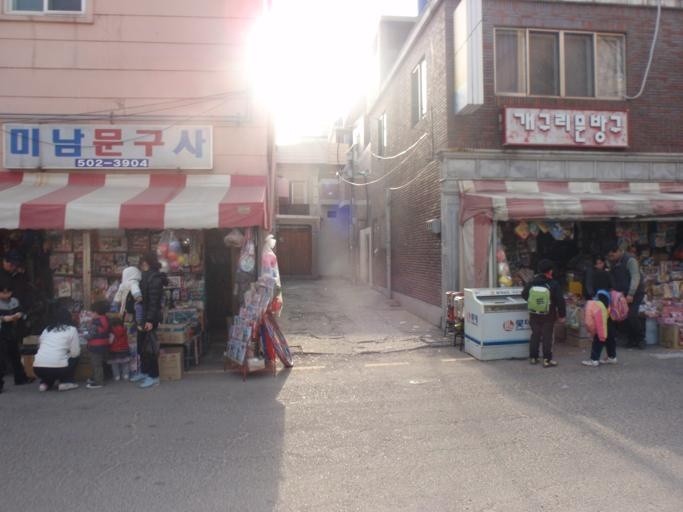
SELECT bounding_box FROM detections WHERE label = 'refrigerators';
[463,288,554,361]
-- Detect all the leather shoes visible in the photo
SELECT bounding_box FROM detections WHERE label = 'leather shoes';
[15,378,34,385]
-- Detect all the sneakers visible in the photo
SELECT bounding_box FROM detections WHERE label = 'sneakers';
[39,385,47,393]
[132,373,145,381]
[530,356,540,364]
[58,382,78,390]
[116,374,121,380]
[87,378,97,383]
[86,384,104,389]
[542,358,558,367]
[124,375,129,380]
[581,359,599,366]
[138,376,160,388]
[600,357,617,365]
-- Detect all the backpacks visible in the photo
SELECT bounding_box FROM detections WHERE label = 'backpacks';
[609,289,629,322]
[527,283,551,317]
[594,288,630,323]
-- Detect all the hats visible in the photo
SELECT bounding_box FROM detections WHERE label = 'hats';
[9,251,24,263]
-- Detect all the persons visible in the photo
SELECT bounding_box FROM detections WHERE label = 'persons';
[108,319,131,380]
[582,271,630,367]
[606,245,647,350]
[582,253,617,297]
[0,277,35,393]
[0,249,23,298]
[127,255,163,389]
[17,264,25,273]
[114,267,144,332]
[81,301,109,389]
[32,309,80,392]
[521,258,567,367]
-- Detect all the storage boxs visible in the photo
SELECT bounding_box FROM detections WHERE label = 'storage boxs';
[158,349,185,382]
[74,348,92,377]
[155,322,189,346]
[567,334,591,351]
[660,324,682,349]
[21,336,39,379]
